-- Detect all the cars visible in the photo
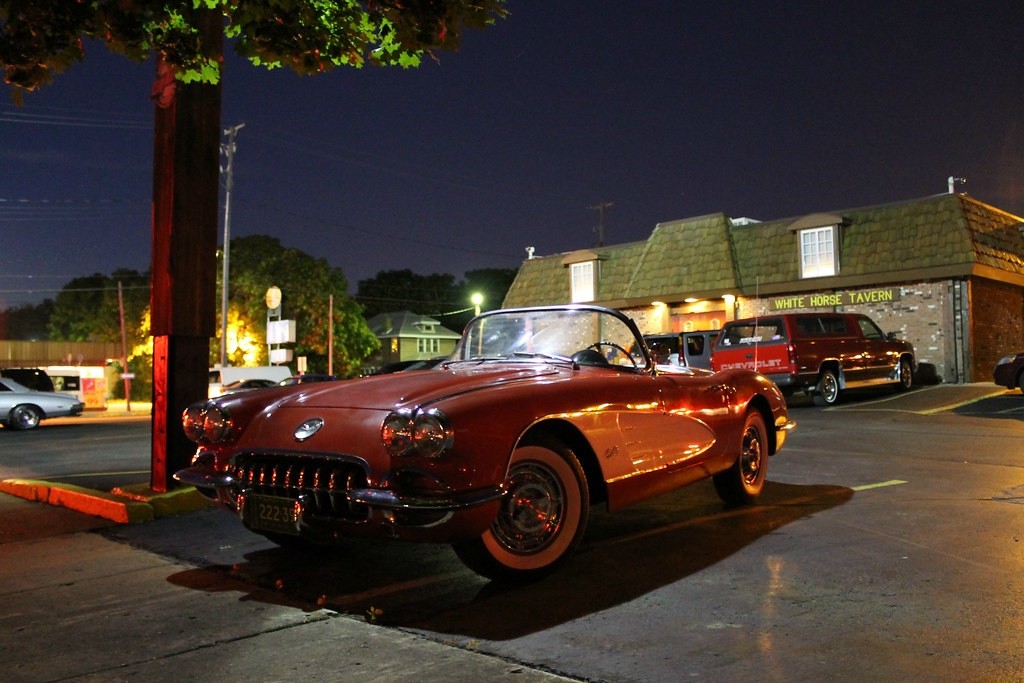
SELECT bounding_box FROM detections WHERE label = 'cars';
[0,375,86,432]
[216,373,337,396]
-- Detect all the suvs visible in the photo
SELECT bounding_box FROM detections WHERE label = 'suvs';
[709,312,919,408]
[618,328,723,370]
[0,367,56,393]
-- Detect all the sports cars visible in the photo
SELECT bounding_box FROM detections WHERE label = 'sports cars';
[171,304,805,592]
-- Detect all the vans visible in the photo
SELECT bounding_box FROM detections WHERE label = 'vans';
[207,364,293,401]
